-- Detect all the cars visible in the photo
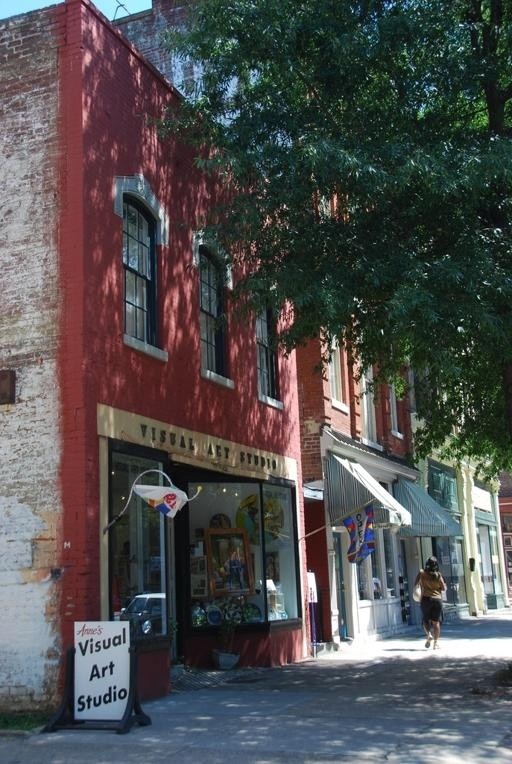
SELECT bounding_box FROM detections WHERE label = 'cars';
[120,593,166,634]
[213,571,224,588]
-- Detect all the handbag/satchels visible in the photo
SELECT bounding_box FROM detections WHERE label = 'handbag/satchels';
[412,572,423,604]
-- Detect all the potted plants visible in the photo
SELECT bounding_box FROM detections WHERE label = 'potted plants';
[212,616,244,670]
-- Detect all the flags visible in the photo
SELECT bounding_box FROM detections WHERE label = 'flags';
[342,501,378,565]
[129,484,189,521]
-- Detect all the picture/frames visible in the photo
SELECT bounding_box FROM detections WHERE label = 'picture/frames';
[203,525,256,598]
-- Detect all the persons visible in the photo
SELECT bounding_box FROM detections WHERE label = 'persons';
[414,556,447,649]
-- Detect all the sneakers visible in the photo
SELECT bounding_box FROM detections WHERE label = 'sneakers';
[424,635,442,650]
